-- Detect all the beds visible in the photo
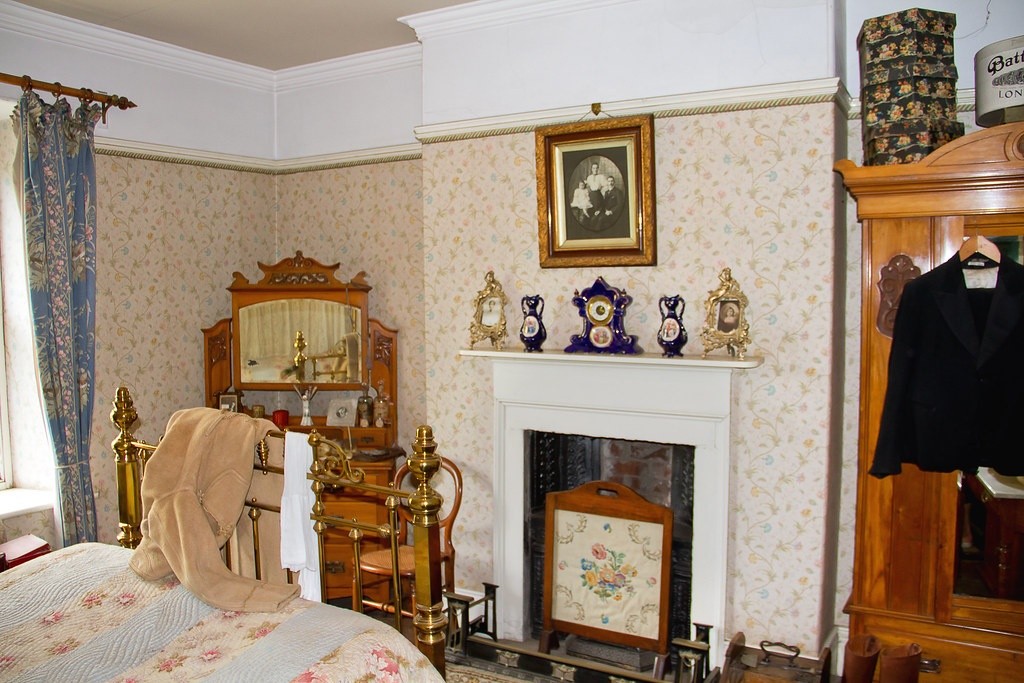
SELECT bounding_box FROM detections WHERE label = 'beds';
[0,387,449,683]
[246,330,361,382]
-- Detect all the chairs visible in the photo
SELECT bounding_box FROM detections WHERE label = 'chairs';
[352,458,463,647]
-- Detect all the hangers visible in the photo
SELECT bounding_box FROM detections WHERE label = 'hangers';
[959,217,1001,263]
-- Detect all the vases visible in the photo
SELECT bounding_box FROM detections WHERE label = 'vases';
[357,384,389,425]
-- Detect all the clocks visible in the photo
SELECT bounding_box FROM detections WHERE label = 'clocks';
[563,276,645,355]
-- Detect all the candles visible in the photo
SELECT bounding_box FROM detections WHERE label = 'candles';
[273,410,289,427]
[253,405,265,418]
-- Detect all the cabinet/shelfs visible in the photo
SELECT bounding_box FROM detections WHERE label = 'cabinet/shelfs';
[833,121,1024,683]
[201,250,404,604]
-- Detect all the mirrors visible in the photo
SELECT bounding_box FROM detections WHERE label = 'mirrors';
[951,233,1024,603]
[239,297,364,384]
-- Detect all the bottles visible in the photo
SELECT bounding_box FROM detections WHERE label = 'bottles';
[356,381,389,428]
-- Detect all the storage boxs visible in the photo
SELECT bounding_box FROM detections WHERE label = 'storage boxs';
[856,7,965,166]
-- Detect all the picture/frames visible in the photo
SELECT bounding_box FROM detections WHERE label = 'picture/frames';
[534,114,657,268]
[218,392,241,413]
[326,399,358,427]
